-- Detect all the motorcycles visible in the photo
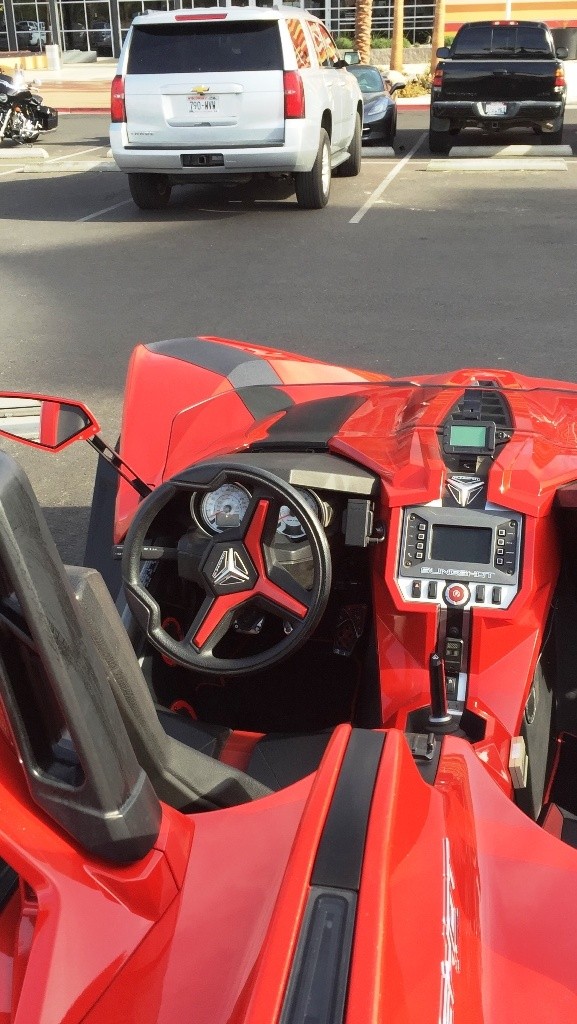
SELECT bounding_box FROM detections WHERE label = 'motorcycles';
[0,64,59,147]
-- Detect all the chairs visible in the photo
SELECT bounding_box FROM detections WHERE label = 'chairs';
[0,446,281,859]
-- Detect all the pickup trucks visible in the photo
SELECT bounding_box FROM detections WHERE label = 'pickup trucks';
[429,21,570,153]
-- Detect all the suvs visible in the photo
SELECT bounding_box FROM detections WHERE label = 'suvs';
[109,5,364,209]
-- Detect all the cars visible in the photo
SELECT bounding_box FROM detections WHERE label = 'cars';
[345,64,405,140]
[90,32,111,47]
[93,23,106,37]
[16,21,46,46]
